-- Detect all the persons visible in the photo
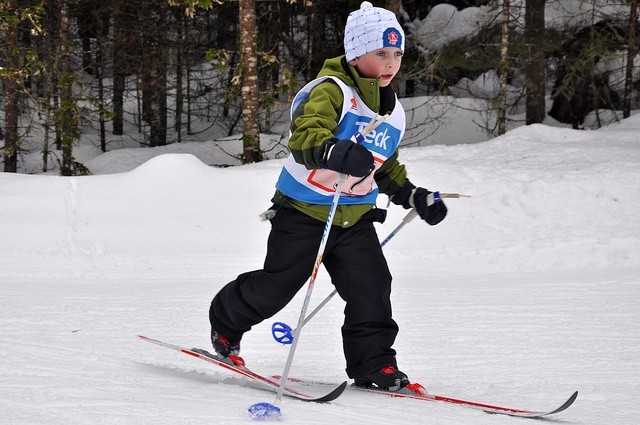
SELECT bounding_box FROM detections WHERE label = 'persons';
[210,2,450,392]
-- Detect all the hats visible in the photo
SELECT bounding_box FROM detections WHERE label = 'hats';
[344,1,406,63]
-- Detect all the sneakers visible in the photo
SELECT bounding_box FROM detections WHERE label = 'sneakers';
[209,324,241,360]
[355,364,411,392]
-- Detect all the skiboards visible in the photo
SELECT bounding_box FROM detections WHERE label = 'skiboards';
[138,337,578,418]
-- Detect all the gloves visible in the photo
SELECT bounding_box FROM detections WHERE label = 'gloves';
[315,138,375,177]
[388,177,448,227]
